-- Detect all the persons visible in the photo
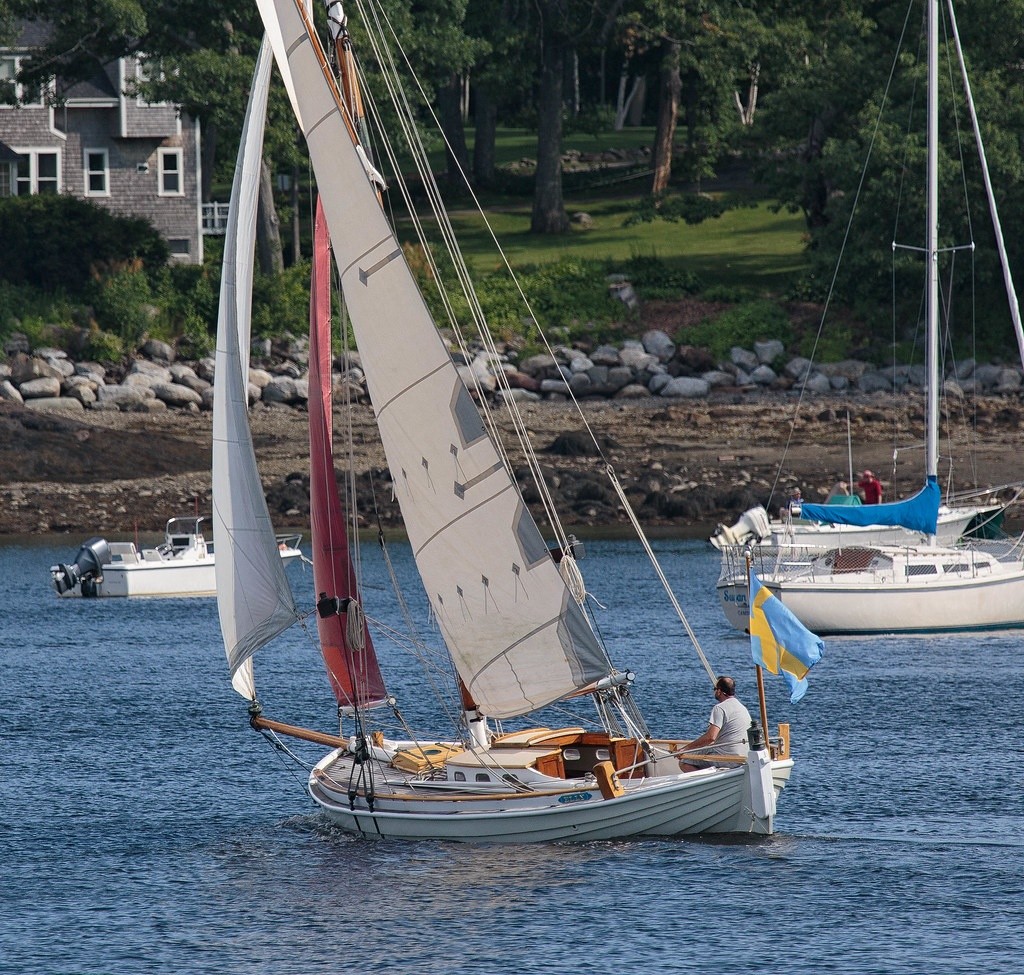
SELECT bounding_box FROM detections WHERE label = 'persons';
[847,470,882,505]
[785,488,804,511]
[675,676,753,773]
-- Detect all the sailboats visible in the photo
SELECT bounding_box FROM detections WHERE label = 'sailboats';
[704,0,1024,636]
[201,0,792,846]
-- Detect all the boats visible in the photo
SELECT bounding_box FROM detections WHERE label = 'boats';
[53,516,308,601]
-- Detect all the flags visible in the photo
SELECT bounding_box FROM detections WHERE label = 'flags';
[747,565,825,704]
